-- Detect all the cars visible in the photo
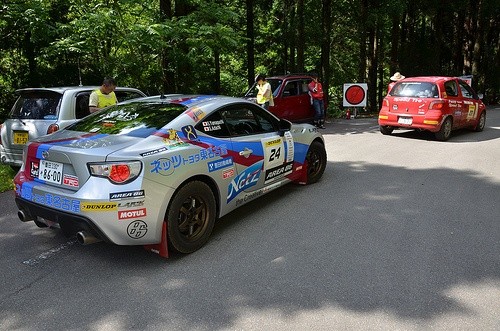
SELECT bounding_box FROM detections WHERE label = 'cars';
[242,75,327,124]
[0,86,148,171]
[12,94,328,256]
[378,76,487,142]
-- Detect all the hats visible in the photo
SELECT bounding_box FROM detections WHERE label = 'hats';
[390,72,405,81]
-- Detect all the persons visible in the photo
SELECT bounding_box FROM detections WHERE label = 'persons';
[88,77,119,115]
[254,73,274,110]
[387,72,405,94]
[308,73,326,130]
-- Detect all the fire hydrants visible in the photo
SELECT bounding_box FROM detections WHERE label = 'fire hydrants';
[346,109,351,119]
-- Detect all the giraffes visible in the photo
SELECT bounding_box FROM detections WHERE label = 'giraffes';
[162,128,184,147]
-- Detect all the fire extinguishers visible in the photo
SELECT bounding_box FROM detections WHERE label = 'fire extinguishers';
[346,108,351,120]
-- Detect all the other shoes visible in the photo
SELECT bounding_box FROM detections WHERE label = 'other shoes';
[314,120,326,129]
